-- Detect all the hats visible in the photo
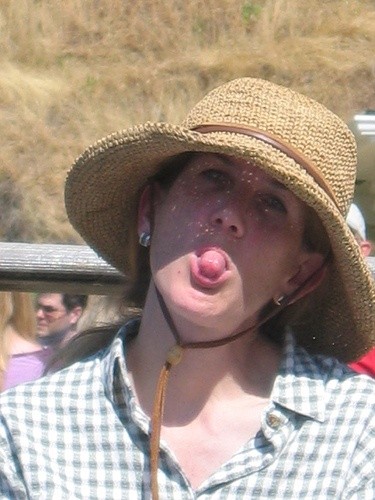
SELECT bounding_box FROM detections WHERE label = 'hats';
[63,76,375,364]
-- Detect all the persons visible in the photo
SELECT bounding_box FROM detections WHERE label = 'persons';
[0,75,375,500]
[319,196,375,378]
[0,283,49,392]
[34,287,89,366]
